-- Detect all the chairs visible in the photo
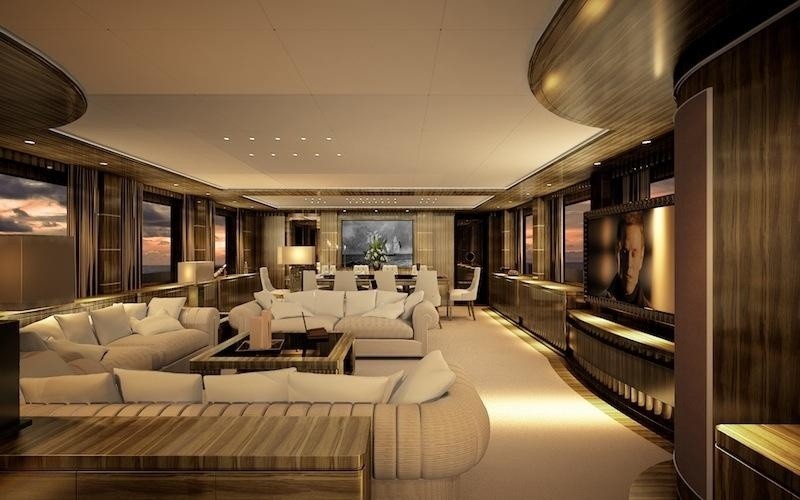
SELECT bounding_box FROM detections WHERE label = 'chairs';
[260,266,276,291]
[302,264,482,321]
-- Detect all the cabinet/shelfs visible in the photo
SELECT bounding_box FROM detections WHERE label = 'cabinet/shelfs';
[1,414,374,500]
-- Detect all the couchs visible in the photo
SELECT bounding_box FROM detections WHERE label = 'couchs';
[227,296,441,358]
[18,306,221,373]
[20,358,491,500]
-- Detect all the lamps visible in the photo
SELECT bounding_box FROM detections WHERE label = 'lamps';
[177,260,216,308]
[276,245,317,293]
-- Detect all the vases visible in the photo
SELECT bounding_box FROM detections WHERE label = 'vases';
[372,260,381,271]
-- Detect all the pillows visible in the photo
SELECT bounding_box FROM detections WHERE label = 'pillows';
[19,294,189,376]
[253,289,313,320]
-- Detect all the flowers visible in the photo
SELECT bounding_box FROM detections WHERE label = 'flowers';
[363,231,391,268]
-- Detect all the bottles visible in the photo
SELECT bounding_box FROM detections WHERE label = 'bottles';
[243,261,249,276]
[213,263,227,278]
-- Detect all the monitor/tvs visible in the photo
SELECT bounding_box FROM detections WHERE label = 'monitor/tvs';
[583,194,675,326]
